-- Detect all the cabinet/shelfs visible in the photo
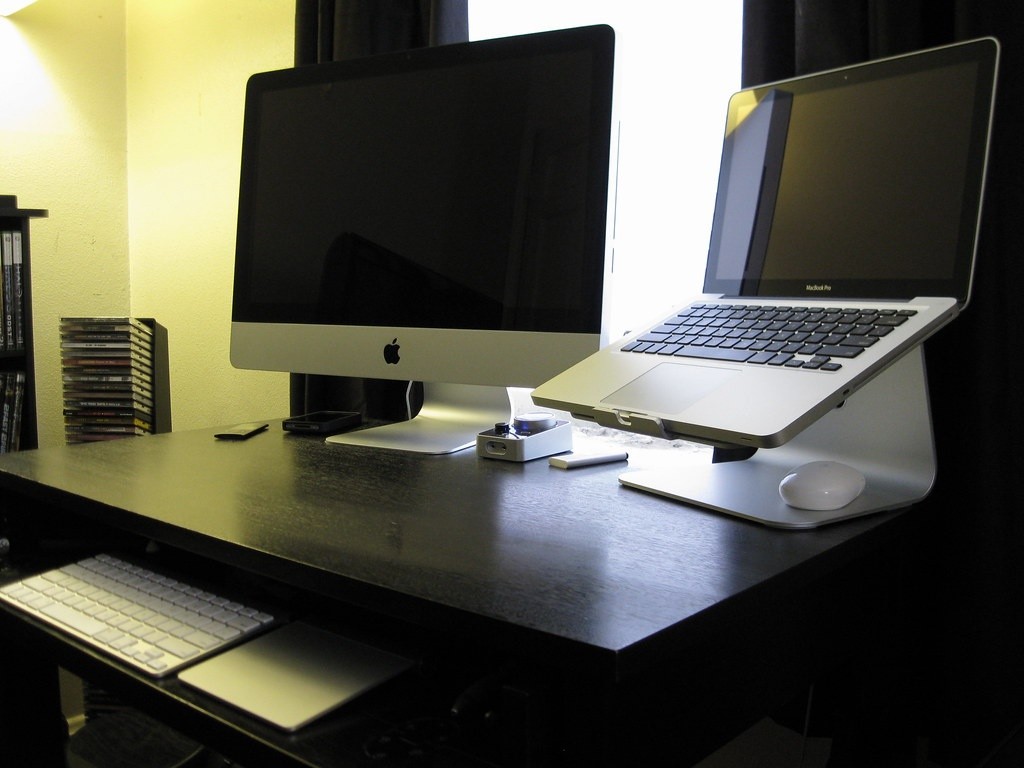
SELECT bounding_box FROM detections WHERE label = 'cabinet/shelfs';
[0,188,50,450]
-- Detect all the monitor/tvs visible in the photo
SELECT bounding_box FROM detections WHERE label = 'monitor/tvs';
[232,22,615,455]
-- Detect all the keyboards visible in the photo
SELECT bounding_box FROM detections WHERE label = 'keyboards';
[0,552,277,681]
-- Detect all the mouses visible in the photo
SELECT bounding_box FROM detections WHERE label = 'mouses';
[780,461,867,511]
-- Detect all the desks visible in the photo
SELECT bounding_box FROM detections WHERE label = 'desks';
[0,401,953,768]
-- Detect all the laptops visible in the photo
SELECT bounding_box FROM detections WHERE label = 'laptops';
[529,34,1000,444]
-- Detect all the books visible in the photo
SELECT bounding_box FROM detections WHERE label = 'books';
[0,229,26,352]
[59,316,154,443]
[0,369,27,453]
